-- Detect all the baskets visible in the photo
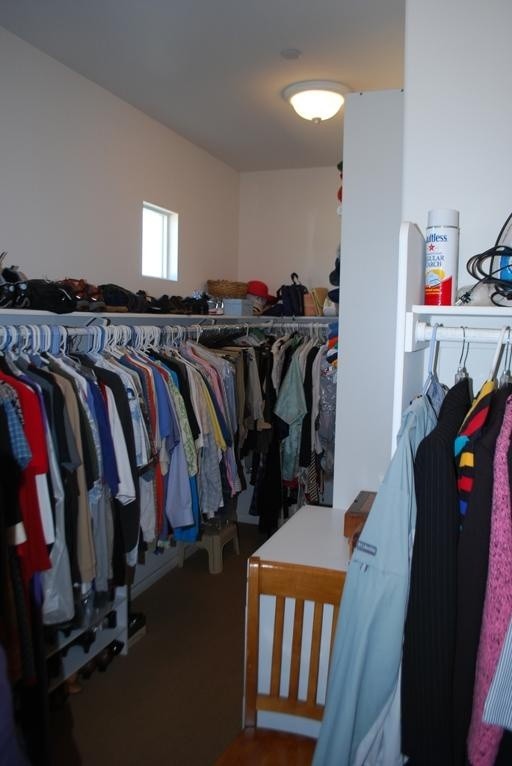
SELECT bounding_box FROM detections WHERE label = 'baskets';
[207,279,247,299]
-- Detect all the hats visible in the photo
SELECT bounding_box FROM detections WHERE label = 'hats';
[248,281,271,298]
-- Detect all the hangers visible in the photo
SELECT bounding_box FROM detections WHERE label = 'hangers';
[421,322,512,397]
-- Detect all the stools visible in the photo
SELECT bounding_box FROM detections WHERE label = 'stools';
[176,522,240,574]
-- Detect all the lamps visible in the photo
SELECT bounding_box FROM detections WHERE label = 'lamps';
[279,80,353,125]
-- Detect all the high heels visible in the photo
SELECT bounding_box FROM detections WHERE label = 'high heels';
[45,587,122,693]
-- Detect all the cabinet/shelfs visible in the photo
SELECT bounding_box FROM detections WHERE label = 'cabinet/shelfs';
[32,584,129,713]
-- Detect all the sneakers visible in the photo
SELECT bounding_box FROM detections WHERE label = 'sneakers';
[1,267,224,315]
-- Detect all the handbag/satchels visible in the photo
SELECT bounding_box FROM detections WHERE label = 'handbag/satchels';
[304,287,328,316]
[280,285,307,316]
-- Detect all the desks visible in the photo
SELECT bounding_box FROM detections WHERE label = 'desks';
[241,504,350,741]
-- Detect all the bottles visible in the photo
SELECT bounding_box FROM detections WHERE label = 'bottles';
[424,208,459,307]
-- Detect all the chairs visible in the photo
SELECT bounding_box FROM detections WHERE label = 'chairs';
[211,556,347,766]
